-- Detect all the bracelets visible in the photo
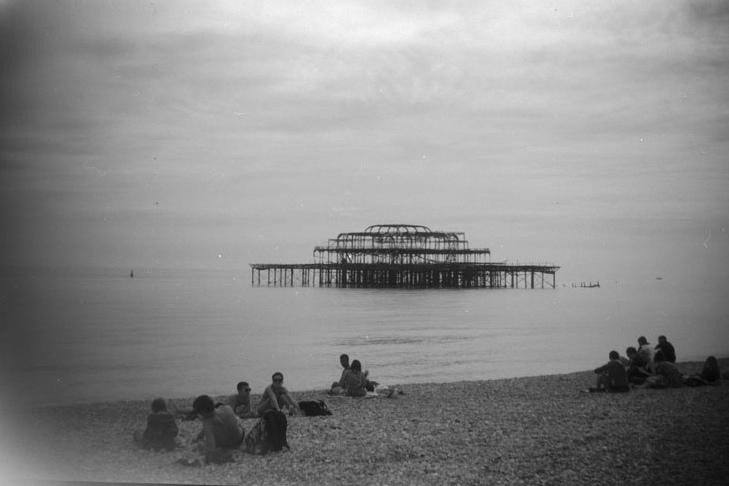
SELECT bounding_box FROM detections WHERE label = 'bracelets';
[280,409,281,413]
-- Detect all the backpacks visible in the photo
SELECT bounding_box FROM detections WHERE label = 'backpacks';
[244,408,289,456]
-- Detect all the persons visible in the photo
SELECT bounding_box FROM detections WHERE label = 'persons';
[134,397,178,452]
[255,371,300,417]
[326,354,367,398]
[351,360,403,395]
[192,395,247,452]
[586,334,719,392]
[172,382,258,419]
